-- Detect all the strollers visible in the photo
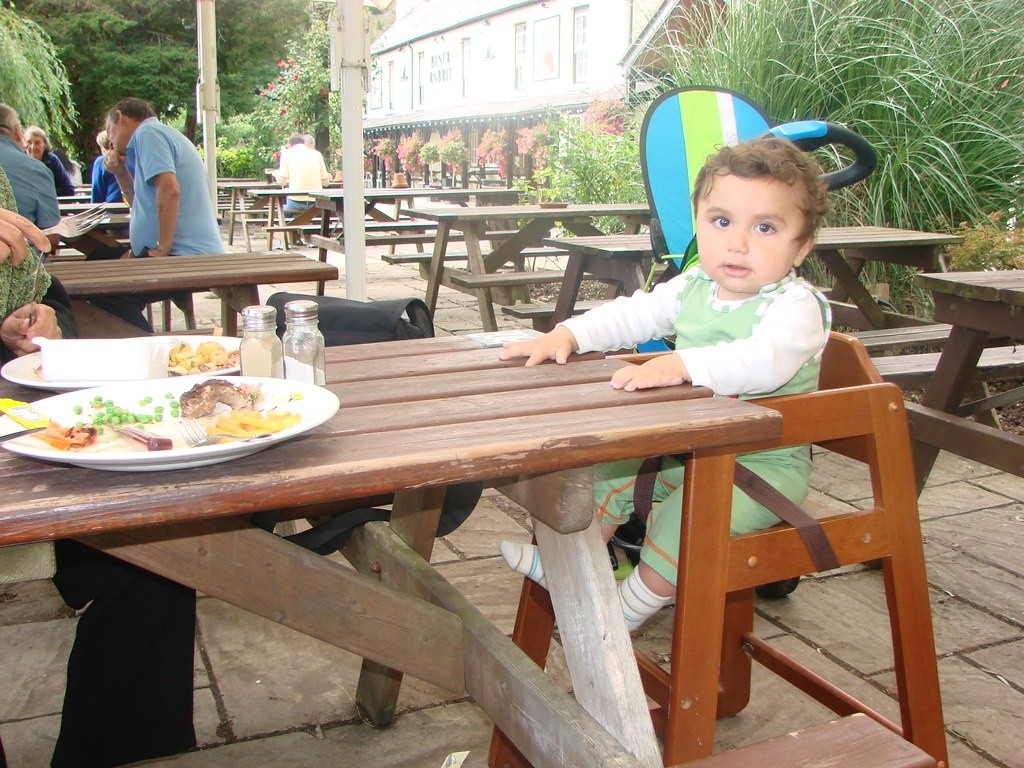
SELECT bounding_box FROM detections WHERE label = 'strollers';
[602,85,879,601]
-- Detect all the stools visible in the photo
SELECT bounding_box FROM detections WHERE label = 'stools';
[489,331,954,768]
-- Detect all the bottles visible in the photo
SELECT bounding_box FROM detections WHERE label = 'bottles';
[239,304,285,379]
[281,299,326,388]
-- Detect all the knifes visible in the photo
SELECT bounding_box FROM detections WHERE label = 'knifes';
[0,426,47,443]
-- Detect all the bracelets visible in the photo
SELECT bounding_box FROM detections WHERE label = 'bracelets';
[120,189,134,194]
[157,242,172,251]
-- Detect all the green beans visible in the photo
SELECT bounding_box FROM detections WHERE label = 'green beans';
[74,392,179,434]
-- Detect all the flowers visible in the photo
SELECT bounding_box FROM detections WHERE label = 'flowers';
[367,91,635,181]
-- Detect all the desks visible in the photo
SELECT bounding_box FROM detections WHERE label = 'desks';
[541,224,964,331]
[217,178,262,181]
[217,182,344,253]
[0,332,784,768]
[861,271,1024,572]
[59,214,132,260]
[310,187,527,296]
[397,203,653,330]
[247,187,437,251]
[43,252,338,341]
[58,202,129,217]
[70,182,93,192]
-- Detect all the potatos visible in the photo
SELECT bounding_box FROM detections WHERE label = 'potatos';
[170,341,226,370]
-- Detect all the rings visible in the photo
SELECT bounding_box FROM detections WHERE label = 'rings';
[106,161,109,166]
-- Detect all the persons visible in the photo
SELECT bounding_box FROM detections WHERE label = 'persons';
[91,130,127,203]
[499,137,831,633]
[0,163,78,368]
[0,104,61,263]
[23,126,82,196]
[274,133,330,250]
[83,98,226,333]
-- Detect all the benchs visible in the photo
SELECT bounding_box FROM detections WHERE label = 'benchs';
[46,193,1024,432]
[667,709,936,768]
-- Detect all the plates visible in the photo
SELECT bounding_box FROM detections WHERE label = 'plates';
[0,375,341,472]
[0,336,271,393]
[539,203,569,208]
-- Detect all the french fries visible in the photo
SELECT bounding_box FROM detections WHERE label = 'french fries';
[205,407,301,438]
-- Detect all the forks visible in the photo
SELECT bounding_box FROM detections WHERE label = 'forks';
[22,201,108,243]
